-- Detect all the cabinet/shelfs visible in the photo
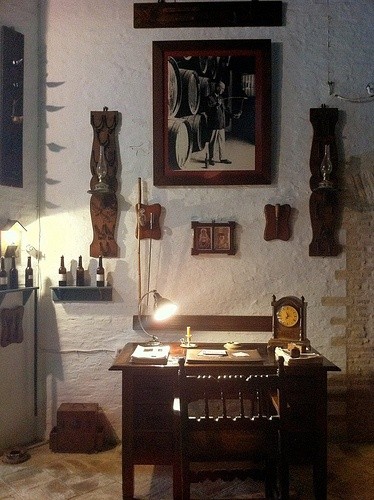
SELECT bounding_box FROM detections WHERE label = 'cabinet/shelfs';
[49,401,106,454]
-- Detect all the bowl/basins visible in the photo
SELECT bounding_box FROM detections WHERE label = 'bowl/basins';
[5,449,27,458]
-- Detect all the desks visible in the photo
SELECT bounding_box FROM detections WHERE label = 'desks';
[109,341,341,499]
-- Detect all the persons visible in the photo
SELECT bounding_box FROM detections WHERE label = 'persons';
[206,82,232,165]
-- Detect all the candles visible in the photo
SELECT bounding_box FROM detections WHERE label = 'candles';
[186,325,191,336]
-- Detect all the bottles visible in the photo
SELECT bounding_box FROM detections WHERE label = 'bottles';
[205,153,209,168]
[0,256,8,291]
[59,256,67,286]
[26,256,33,287]
[76,255,84,286]
[97,256,105,286]
[10,257,18,288]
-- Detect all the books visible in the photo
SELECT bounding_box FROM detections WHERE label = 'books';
[129,344,171,365]
[275,346,322,366]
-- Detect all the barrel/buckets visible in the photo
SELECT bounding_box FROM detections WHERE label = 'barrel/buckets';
[168,55,231,170]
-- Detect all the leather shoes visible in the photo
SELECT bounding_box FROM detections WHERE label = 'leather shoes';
[219,159,232,164]
[209,159,215,165]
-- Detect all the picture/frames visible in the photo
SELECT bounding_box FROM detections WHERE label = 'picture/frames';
[190,221,235,256]
[152,38,272,186]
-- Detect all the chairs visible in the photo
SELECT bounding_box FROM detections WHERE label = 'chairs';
[176,356,283,500]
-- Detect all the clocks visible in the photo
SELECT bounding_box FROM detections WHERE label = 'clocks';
[267,293,311,354]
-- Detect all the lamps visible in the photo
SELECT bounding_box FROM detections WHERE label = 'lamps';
[137,290,178,346]
[1,219,28,257]
